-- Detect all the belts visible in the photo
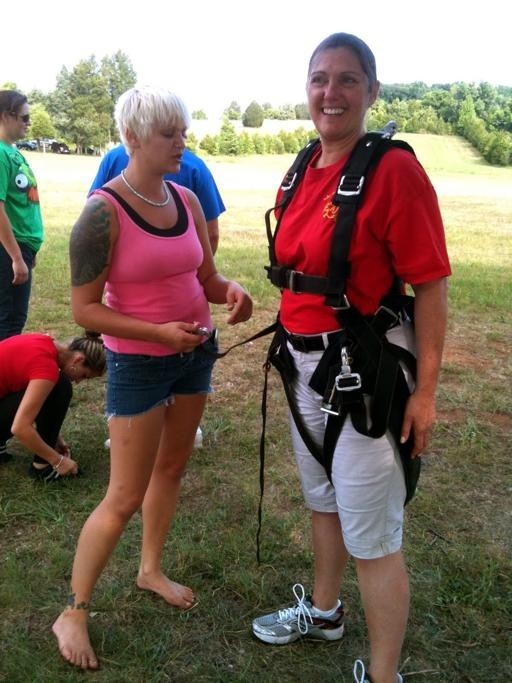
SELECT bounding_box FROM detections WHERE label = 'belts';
[285,331,347,354]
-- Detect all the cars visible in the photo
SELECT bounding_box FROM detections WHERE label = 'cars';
[16,136,101,155]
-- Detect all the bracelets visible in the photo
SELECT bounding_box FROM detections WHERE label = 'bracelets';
[52,456,63,470]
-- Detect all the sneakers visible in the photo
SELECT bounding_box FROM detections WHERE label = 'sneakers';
[29,462,82,482]
[251,592,344,645]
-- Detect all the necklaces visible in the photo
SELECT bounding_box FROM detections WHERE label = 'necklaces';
[121,168,171,206]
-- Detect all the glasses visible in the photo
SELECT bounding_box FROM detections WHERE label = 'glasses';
[6,110,30,123]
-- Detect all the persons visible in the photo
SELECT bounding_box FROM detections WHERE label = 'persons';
[0,90,44,342]
[51,87,253,670]
[86,141,227,451]
[0,328,107,482]
[251,33,453,683]
